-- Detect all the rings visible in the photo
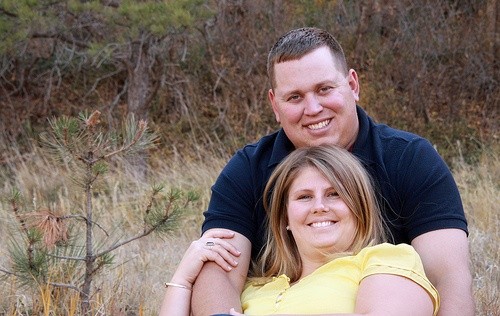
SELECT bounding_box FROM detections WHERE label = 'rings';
[206,241,214,246]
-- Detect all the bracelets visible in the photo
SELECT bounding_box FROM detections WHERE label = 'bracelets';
[164,283,193,291]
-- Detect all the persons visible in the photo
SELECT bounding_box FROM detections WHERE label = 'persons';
[191,27,476,316]
[159,142,441,316]
